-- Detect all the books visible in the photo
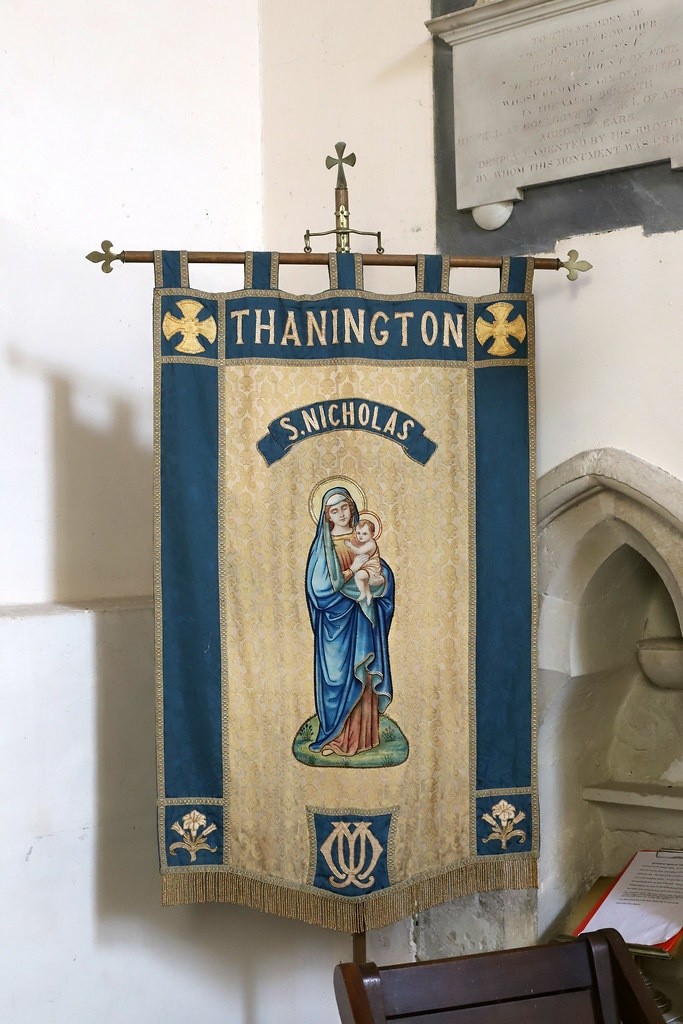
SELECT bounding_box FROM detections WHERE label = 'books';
[557,875,683,959]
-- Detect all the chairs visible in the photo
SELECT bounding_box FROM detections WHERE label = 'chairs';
[333,930,615,1024]
